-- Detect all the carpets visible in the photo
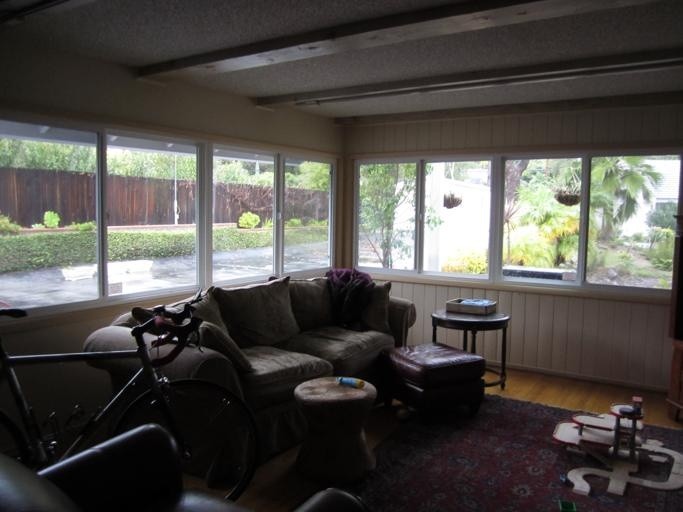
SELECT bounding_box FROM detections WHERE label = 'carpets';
[345,393,683,512]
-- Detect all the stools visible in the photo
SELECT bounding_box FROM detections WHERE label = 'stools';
[291,311,510,490]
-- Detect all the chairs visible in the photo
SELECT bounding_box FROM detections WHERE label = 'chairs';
[0,423,371,511]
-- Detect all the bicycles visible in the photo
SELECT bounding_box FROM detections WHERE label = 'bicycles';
[0,284,257,506]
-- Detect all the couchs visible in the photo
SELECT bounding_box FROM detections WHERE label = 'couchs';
[81,274,415,476]
